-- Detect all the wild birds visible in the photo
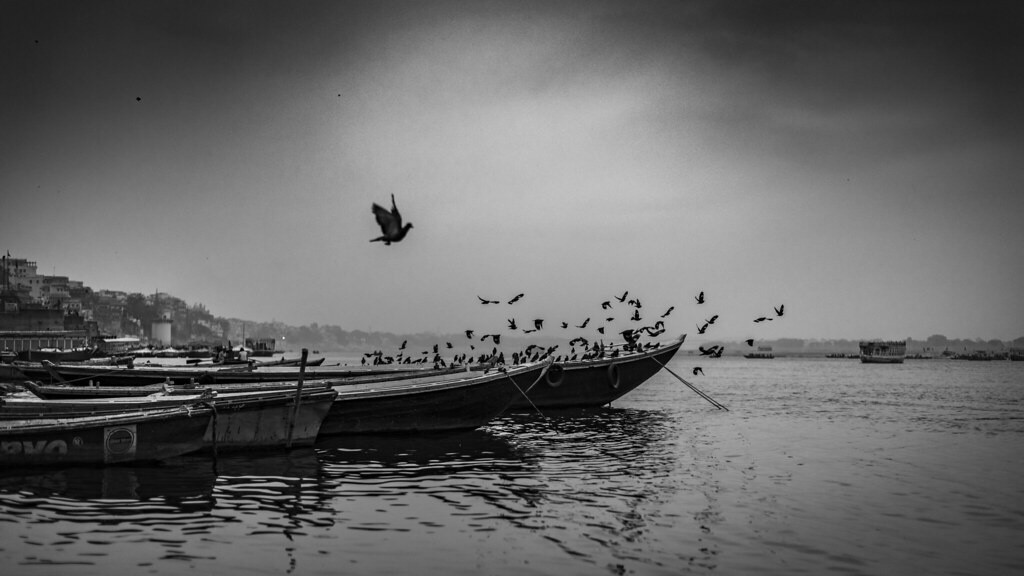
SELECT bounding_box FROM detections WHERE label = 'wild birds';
[620,321,665,352]
[575,317,590,329]
[564,336,619,361]
[513,345,558,366]
[706,315,719,324]
[607,318,614,321]
[695,291,705,304]
[446,342,452,348]
[523,330,537,334]
[368,193,414,245]
[699,345,724,358]
[600,300,612,309]
[597,326,604,334]
[693,366,705,376]
[695,323,708,334]
[660,306,675,317]
[533,320,544,330]
[626,298,642,309]
[508,294,524,304]
[774,304,784,316]
[753,317,773,322]
[614,292,628,302]
[561,322,568,328]
[465,329,474,339]
[745,338,754,346]
[477,295,499,305]
[507,318,517,329]
[630,309,643,321]
[450,344,506,365]
[361,340,447,370]
[481,334,501,345]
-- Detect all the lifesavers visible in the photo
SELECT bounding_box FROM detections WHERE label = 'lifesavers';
[545,363,565,388]
[608,362,621,390]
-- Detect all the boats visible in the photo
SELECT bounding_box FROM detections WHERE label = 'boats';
[174,357,325,368]
[22,355,556,434]
[18,345,98,361]
[859,340,907,363]
[1010,347,1024,361]
[41,332,688,411]
[744,353,775,359]
[826,353,846,358]
[848,354,859,359]
[0,388,218,472]
[0,387,341,452]
